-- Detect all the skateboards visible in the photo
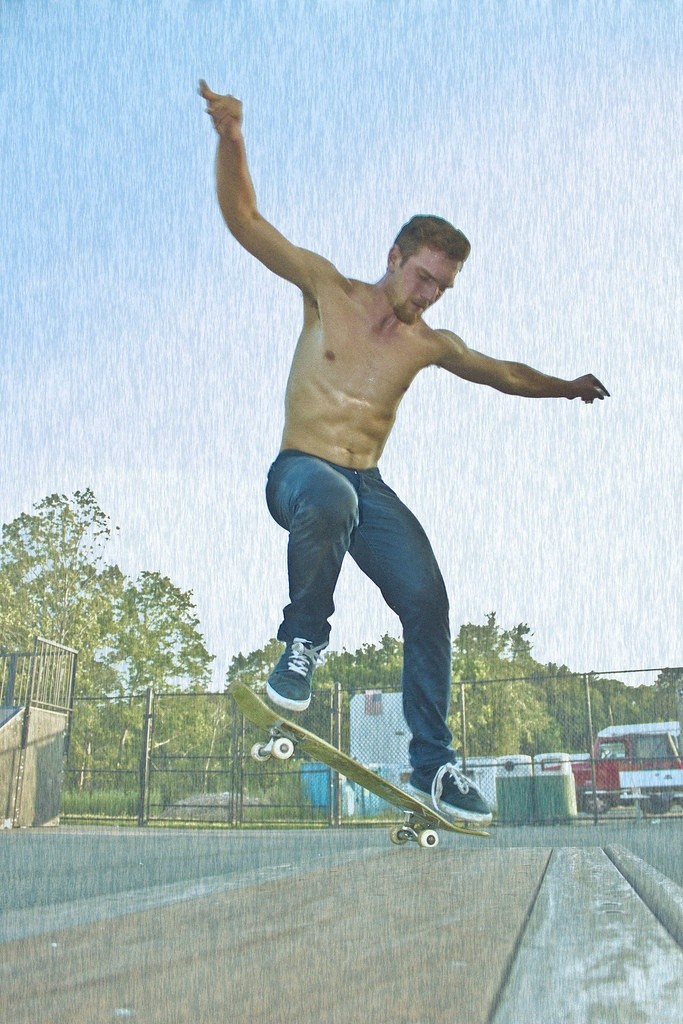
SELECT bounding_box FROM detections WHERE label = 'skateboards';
[230,681,492,850]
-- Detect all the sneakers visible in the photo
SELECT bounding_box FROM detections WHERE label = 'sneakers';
[408,765,493,822]
[267,637,329,711]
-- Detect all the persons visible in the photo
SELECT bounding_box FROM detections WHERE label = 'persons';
[199,81,609,819]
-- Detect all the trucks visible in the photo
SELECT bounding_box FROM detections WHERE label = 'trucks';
[547,719,683,816]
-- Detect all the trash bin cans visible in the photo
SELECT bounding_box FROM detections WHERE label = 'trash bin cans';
[534,753,578,824]
[495,754,535,825]
